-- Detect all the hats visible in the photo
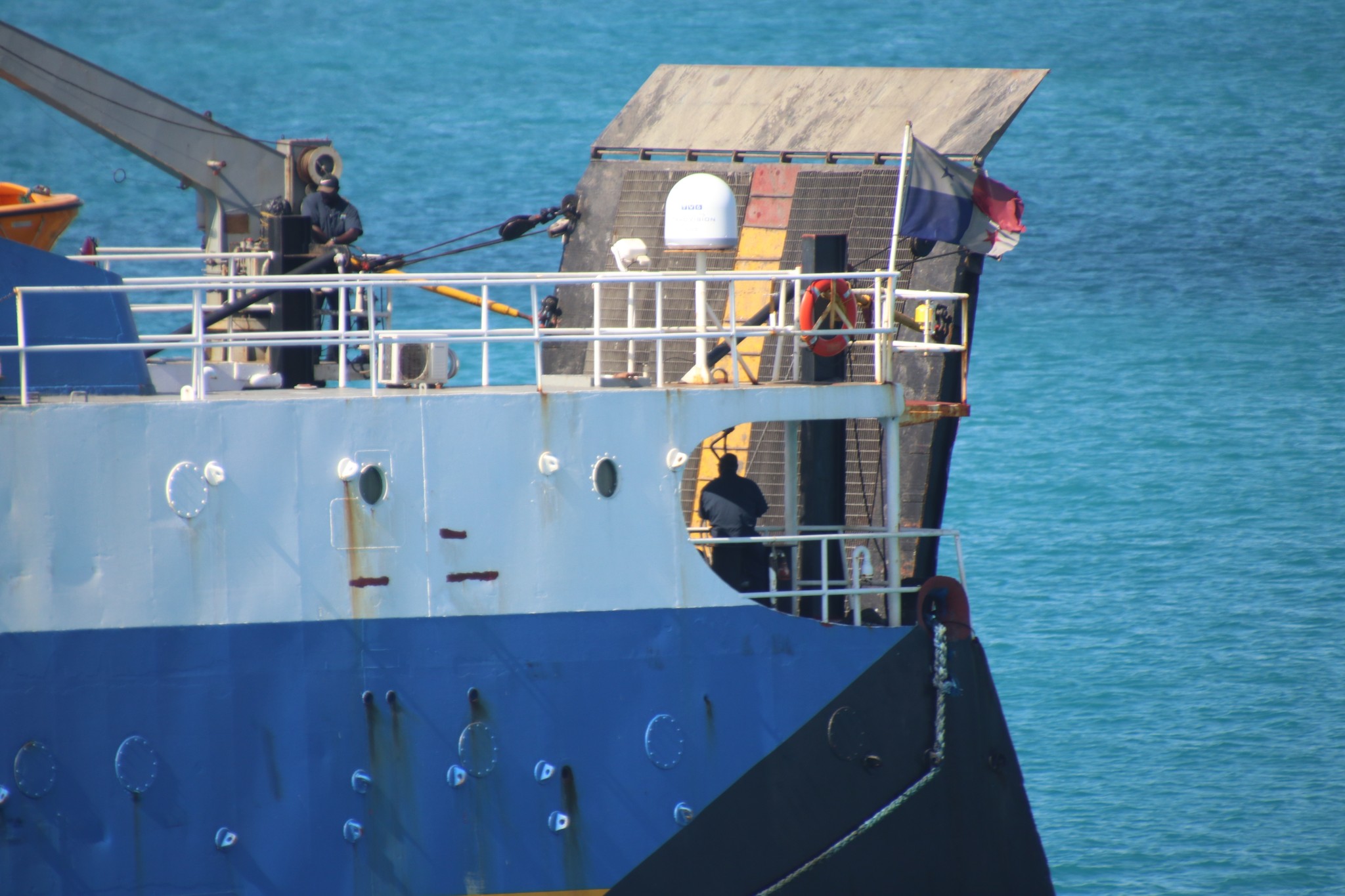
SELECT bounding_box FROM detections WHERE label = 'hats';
[316,174,339,194]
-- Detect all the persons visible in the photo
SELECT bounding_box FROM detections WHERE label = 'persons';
[300,174,363,365]
[699,453,767,602]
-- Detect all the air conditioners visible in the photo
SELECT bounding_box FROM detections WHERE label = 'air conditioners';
[377,333,449,391]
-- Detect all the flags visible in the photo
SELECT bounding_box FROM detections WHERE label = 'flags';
[897,140,1027,261]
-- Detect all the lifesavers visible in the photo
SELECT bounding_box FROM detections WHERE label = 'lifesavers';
[799,278,858,356]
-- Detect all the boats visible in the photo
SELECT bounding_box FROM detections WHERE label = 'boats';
[0,14,1054,896]
